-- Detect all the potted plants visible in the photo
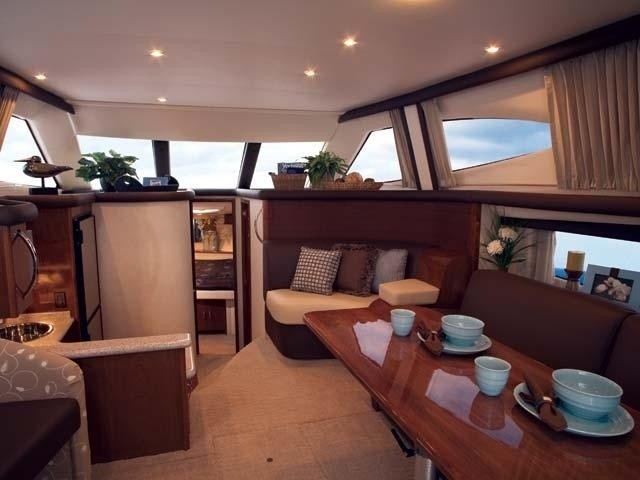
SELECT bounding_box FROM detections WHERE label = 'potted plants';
[75,149,139,191]
[296,152,348,188]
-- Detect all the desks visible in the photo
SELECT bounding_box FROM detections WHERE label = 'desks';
[302,299,640,480]
[0,399,81,480]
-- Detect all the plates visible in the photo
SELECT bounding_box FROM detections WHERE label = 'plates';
[442,335,492,354]
[514,382,635,437]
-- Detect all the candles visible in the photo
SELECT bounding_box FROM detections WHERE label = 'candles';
[566,250,584,272]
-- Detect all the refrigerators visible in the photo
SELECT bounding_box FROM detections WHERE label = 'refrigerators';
[71,214,103,341]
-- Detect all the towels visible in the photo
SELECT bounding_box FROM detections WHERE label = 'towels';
[413,321,446,357]
[519,369,567,432]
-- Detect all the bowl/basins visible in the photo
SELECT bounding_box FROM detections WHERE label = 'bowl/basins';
[552,368,623,420]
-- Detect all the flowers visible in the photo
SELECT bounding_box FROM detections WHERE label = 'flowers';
[473,202,539,273]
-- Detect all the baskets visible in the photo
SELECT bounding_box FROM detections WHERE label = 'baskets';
[313,181,384,192]
[269,172,308,191]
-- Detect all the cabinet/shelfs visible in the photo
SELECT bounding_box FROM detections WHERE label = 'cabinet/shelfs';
[195,299,226,335]
[28,206,103,342]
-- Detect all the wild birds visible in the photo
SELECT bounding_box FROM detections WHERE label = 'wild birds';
[13,155,73,189]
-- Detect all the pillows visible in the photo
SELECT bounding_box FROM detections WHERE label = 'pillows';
[332,243,378,298]
[370,248,409,295]
[291,246,343,296]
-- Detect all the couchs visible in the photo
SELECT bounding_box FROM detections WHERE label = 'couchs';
[262,240,472,360]
[459,268,640,413]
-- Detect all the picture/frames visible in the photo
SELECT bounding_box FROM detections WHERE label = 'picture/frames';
[583,264,640,311]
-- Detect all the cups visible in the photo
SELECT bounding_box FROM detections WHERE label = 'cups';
[441,314,485,346]
[474,356,512,396]
[390,309,416,337]
